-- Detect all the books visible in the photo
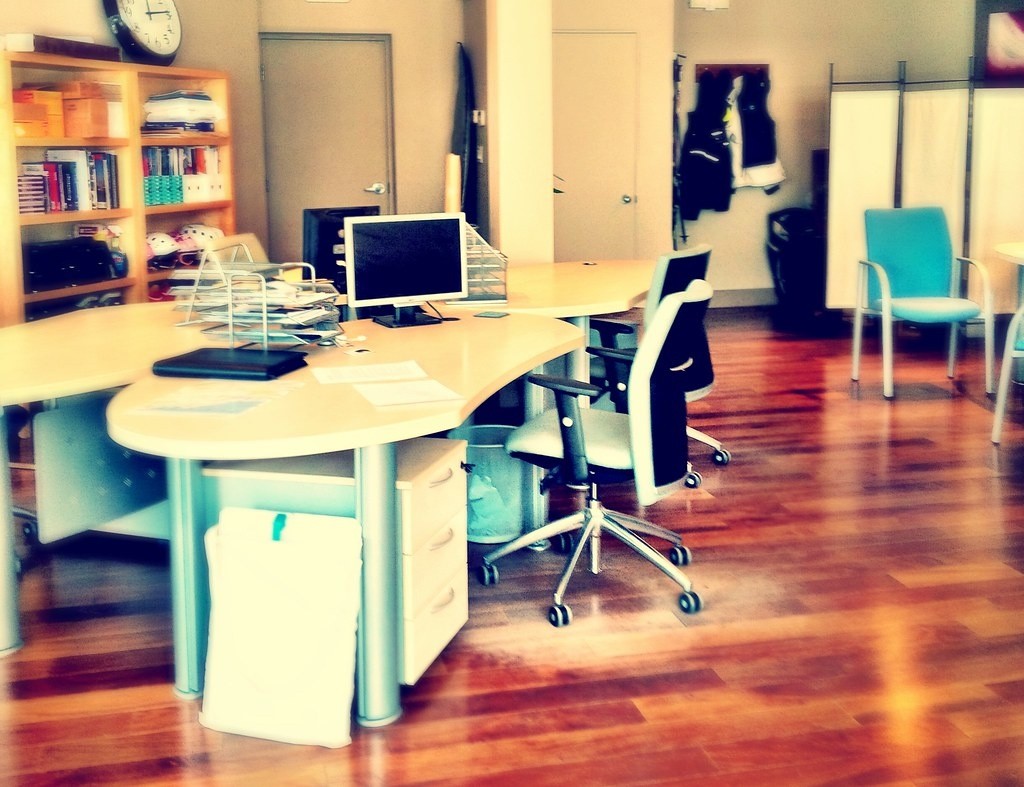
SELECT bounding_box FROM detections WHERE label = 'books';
[21,150,119,212]
[142,145,219,176]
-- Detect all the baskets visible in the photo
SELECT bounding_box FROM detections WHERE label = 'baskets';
[144,174,183,205]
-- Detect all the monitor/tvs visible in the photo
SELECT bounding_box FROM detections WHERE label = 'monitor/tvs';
[301,204,380,294]
[343,211,469,331]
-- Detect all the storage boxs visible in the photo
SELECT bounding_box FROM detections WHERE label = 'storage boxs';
[13,81,125,139]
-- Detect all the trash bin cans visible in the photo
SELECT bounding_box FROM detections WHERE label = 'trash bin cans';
[447,423,523,544]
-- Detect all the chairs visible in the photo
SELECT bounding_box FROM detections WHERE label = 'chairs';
[851,206,995,401]
[545,246,733,487]
[479,280,713,626]
[208,231,268,263]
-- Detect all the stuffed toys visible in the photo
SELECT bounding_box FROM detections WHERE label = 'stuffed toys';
[144,222,224,262]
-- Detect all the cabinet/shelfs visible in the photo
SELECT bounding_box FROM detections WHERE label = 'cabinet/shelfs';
[0,52,236,328]
[199,439,468,684]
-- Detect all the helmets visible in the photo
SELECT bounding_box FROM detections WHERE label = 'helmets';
[174,224,226,260]
[146,231,181,262]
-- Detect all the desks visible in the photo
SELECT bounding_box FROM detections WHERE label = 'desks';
[988,241,1024,445]
[0,257,657,730]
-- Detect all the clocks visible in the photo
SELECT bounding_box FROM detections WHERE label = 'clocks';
[103,0,182,66]
